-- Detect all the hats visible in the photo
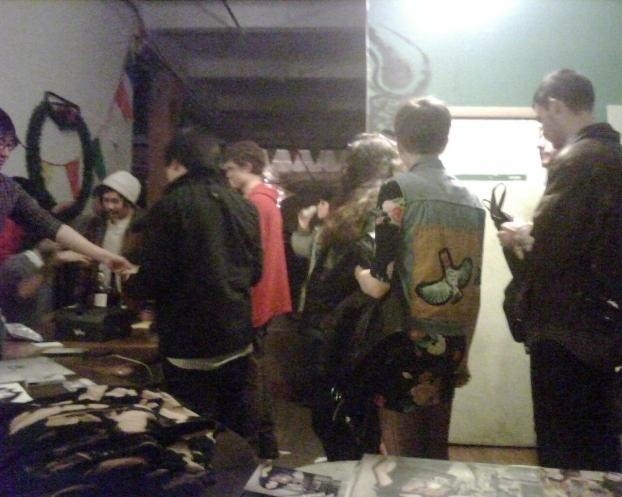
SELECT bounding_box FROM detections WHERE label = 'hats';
[103,170,141,205]
